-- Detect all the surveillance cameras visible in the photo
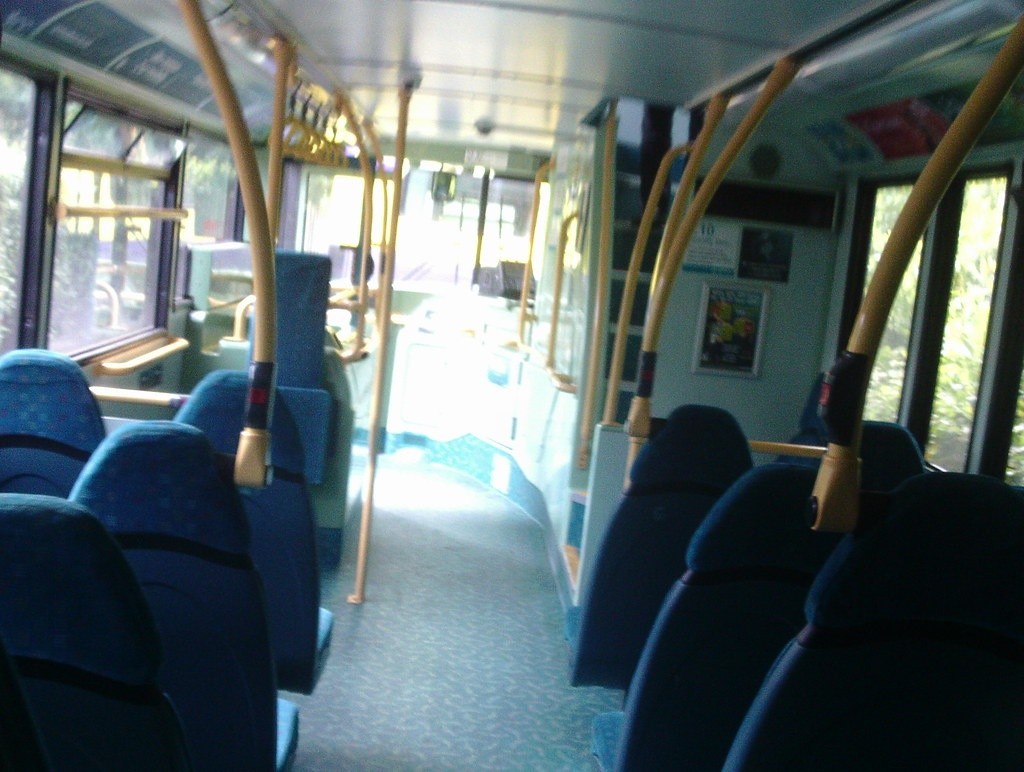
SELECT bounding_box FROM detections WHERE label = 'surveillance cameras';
[475,120,493,135]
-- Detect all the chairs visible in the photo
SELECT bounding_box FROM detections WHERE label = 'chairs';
[0,349,336,772]
[567,405,1024,772]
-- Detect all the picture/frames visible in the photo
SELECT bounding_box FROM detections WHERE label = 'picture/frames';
[693,279,773,379]
